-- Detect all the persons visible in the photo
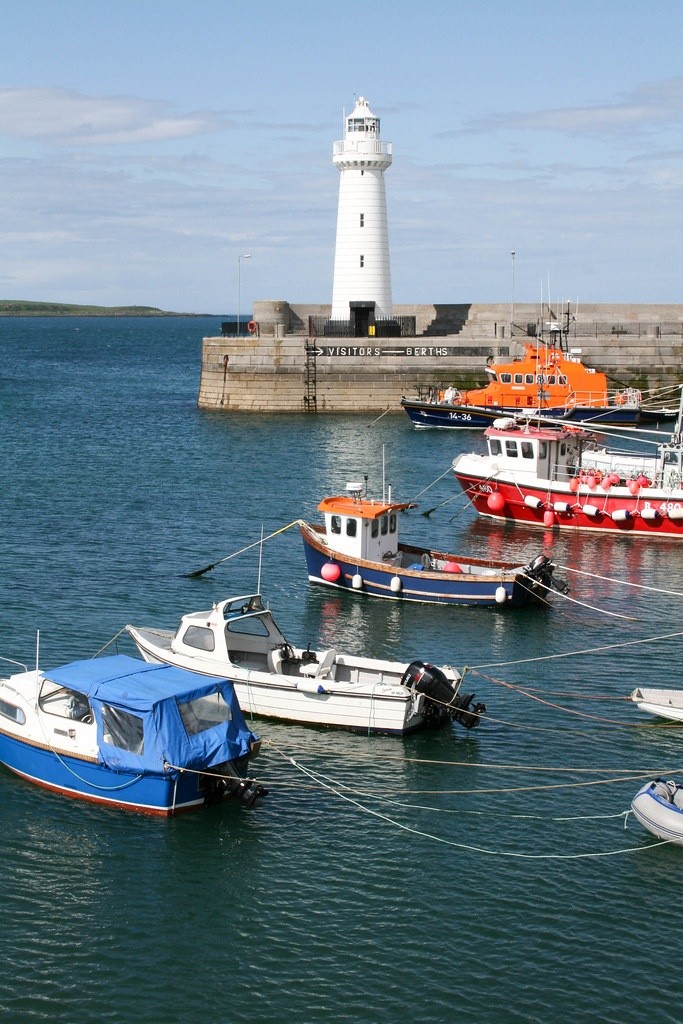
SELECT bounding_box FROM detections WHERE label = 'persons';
[487,354,493,367]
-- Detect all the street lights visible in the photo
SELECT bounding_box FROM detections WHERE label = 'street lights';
[511,250,516,334]
[236,254,251,337]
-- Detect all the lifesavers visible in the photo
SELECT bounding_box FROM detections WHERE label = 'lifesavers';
[248,321,256,332]
[594,471,602,483]
[638,392,642,402]
[579,470,588,481]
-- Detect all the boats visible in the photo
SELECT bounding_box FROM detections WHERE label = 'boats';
[630,776,682,848]
[400,269,643,431]
[0,631,271,819]
[640,407,680,424]
[630,686,682,724]
[451,366,683,539]
[125,522,487,738]
[298,443,570,608]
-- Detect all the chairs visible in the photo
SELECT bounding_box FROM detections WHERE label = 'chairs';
[299,649,336,680]
